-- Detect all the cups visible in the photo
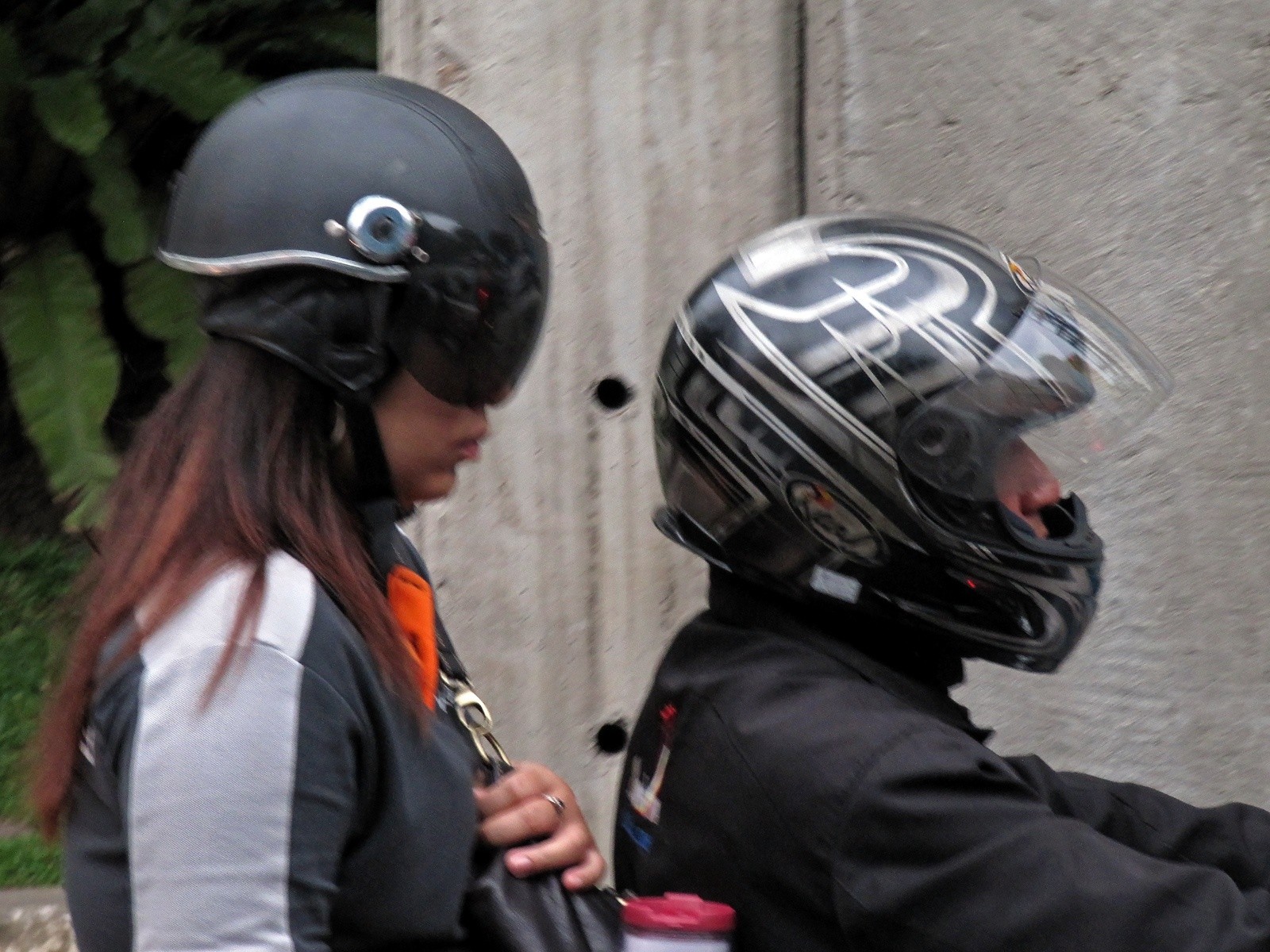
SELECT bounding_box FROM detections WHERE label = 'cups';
[620,889,735,951]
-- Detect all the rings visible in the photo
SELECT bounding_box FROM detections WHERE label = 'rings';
[543,794,565,812]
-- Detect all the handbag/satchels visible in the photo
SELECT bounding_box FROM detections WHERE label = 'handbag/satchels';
[387,522,620,952]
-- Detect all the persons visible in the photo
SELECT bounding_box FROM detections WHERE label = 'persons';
[31,72,606,951]
[613,212,1270,952]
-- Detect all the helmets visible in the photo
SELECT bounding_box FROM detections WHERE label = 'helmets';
[650,214,1105,675]
[156,68,548,402]
